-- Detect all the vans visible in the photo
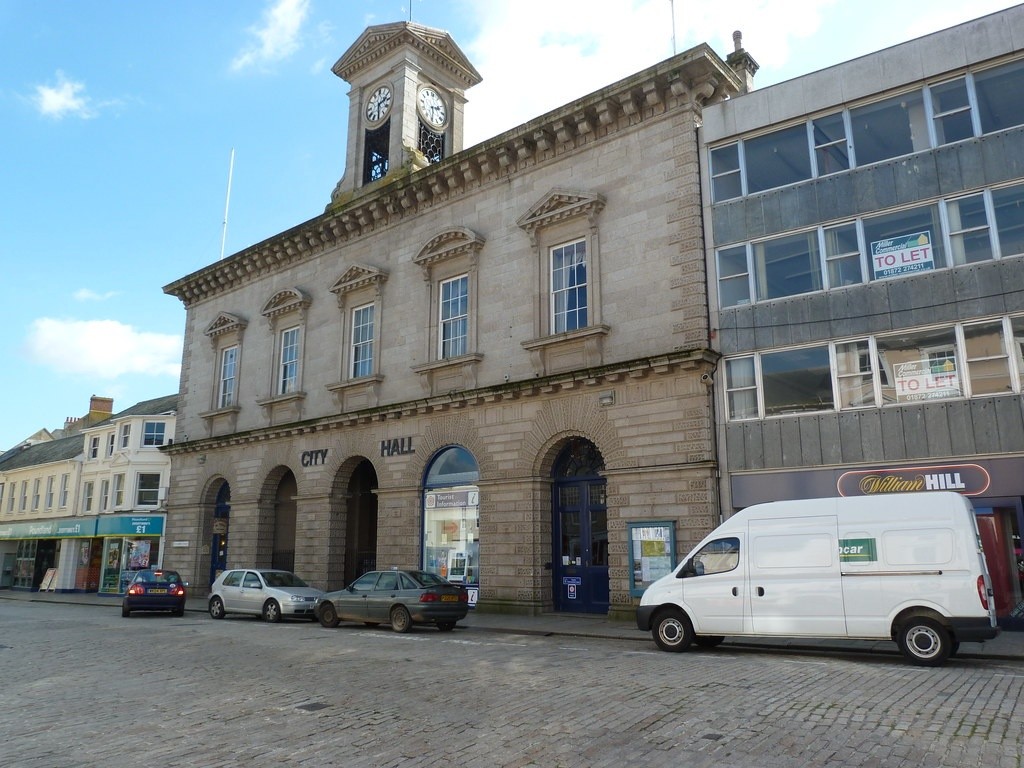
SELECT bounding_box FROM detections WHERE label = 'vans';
[637,491,999,664]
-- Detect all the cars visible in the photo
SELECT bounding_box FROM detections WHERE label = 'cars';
[208,568,328,624]
[313,570,469,634]
[122,570,187,619]
